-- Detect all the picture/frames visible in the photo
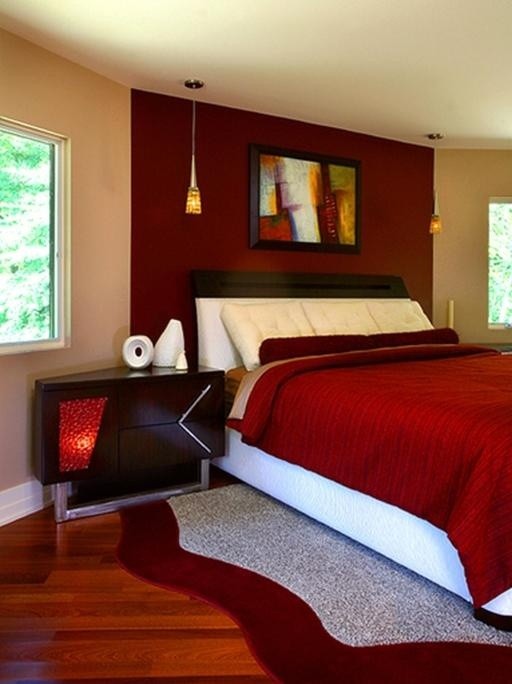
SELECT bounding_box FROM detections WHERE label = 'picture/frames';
[247,141,362,256]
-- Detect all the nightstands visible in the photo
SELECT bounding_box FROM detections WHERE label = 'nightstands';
[32,362,225,523]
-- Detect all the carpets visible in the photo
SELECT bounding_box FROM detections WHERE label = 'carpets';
[114,475,512,684]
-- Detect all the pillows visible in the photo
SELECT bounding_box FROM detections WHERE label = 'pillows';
[220,298,459,373]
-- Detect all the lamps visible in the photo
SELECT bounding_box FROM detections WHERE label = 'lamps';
[184,78,204,215]
[428,132,444,235]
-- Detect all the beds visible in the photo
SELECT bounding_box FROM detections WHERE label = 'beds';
[186,268,512,634]
